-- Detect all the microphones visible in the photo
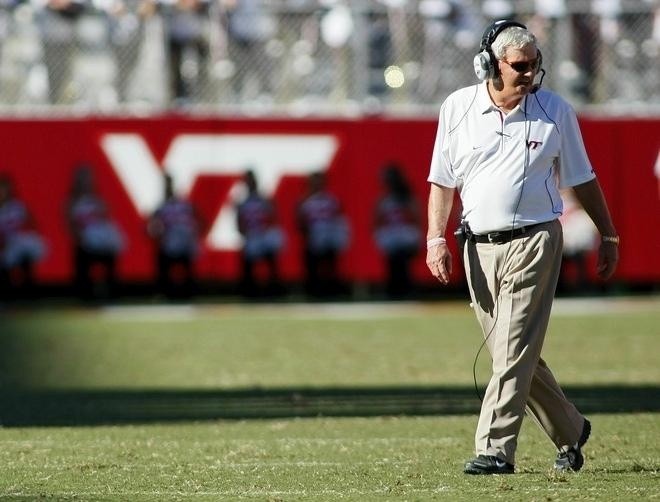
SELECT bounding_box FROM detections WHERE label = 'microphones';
[529,69,546,95]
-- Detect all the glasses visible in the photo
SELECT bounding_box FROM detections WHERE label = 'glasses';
[504,56,541,72]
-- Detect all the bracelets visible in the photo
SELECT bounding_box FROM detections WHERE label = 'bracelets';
[601,236,620,244]
[426,238,447,248]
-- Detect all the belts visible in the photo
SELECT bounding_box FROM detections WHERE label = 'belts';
[468,225,538,244]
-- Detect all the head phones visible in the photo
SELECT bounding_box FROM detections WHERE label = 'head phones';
[473,18,543,81]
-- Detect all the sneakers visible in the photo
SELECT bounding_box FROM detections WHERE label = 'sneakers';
[464,455,514,473]
[554,418,591,470]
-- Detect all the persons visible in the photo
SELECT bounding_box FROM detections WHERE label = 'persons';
[65,165,122,295]
[1,172,43,294]
[145,173,202,293]
[425,19,620,473]
[365,166,425,294]
[297,173,356,295]
[553,163,599,293]
[236,169,286,295]
[32,0,660,104]
